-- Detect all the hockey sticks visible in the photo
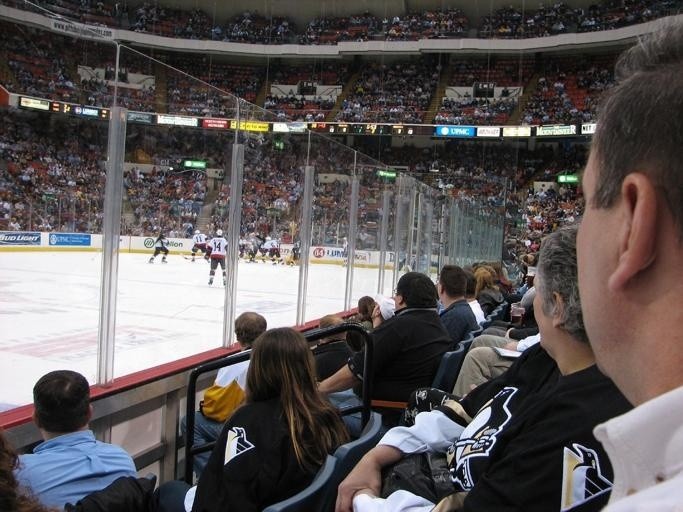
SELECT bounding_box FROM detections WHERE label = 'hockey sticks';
[180,252,206,260]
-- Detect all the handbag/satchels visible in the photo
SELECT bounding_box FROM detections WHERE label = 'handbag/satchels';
[381,450,457,504]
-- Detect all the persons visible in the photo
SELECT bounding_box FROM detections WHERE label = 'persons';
[0,1,682,512]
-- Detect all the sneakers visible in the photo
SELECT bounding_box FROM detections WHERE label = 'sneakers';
[209,279,226,285]
[250,258,293,266]
[149,258,167,264]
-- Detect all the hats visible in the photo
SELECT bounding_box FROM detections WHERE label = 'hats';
[375,293,396,321]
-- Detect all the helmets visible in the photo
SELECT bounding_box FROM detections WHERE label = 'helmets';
[216,228,223,236]
[194,230,200,234]
